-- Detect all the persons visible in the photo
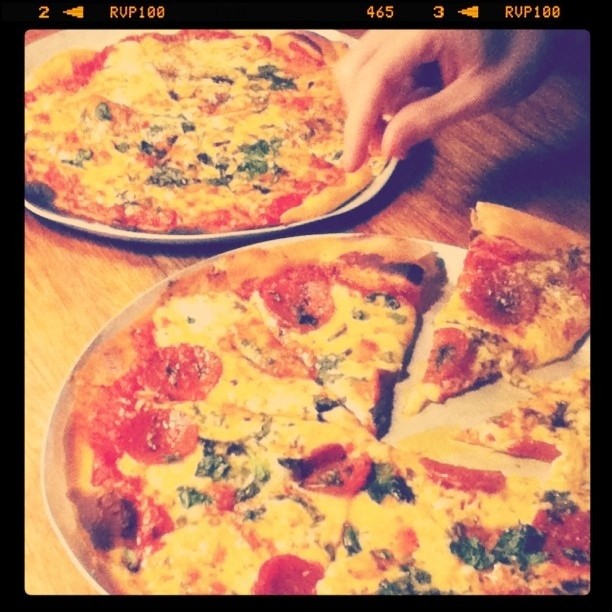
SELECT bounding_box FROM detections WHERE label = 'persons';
[334,29,590,173]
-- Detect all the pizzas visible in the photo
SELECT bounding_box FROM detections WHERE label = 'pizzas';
[395,200,591,423]
